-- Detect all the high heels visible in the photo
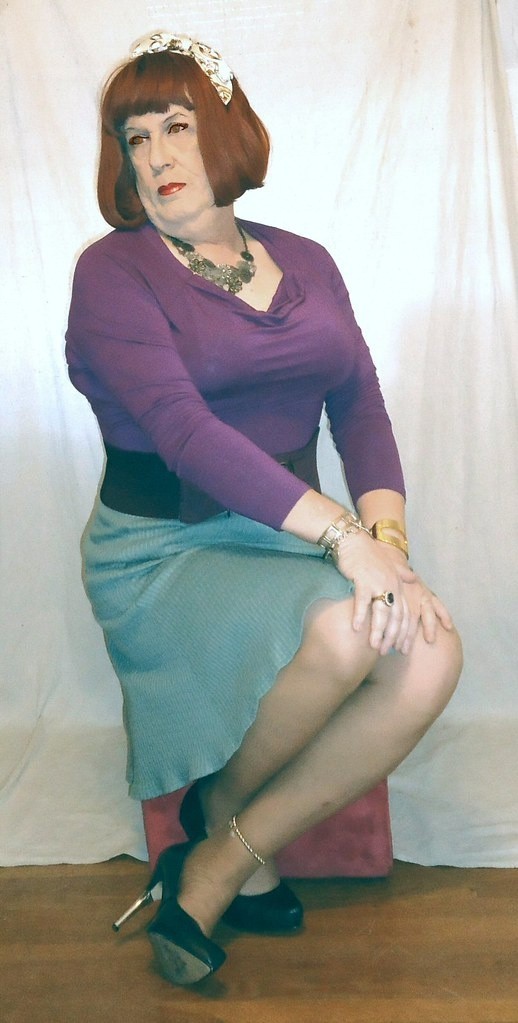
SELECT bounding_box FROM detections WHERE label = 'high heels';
[178,772,305,936]
[112,838,228,988]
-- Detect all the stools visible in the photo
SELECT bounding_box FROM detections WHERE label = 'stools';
[116,671,395,893]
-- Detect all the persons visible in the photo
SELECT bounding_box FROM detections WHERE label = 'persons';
[66,31,464,987]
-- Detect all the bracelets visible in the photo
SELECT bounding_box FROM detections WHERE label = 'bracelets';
[316,510,377,565]
[369,518,410,561]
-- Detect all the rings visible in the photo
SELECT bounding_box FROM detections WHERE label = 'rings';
[371,591,395,606]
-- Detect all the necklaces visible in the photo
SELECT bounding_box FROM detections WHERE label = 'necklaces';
[158,220,256,296]
